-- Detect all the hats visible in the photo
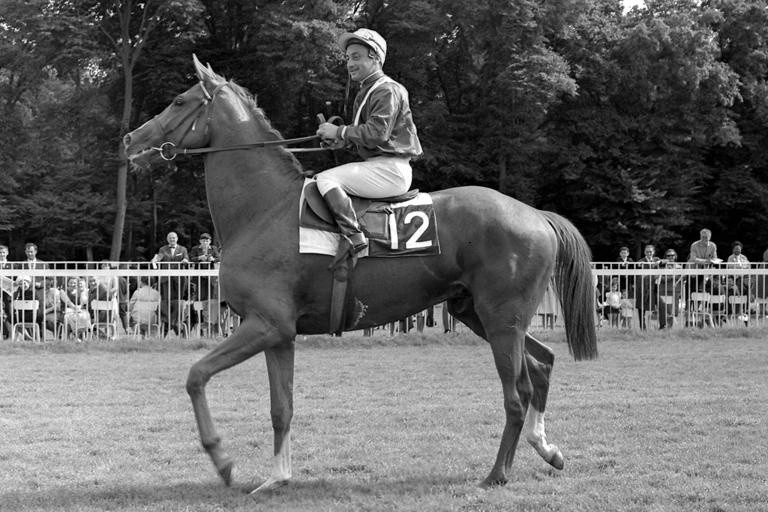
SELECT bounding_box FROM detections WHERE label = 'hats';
[16,275,31,285]
[338,28,387,67]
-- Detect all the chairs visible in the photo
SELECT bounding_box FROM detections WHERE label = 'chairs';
[8,296,236,342]
[688,293,767,328]
[594,293,608,330]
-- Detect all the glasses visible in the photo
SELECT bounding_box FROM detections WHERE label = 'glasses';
[667,255,674,258]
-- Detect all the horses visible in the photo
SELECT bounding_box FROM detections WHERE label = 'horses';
[121,52,600,504]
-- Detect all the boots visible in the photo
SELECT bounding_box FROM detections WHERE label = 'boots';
[323,186,368,271]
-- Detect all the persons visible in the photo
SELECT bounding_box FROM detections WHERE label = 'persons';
[534,228,768,330]
[0,231,220,341]
[442,301,457,333]
[316,28,423,272]
